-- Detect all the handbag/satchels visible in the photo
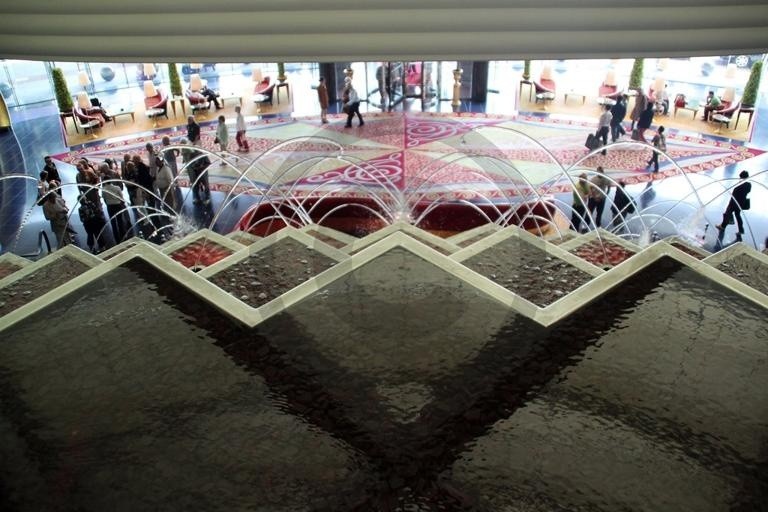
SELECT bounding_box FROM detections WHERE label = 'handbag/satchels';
[657,143,666,154]
[676,100,685,107]
[631,129,640,140]
[626,196,637,213]
[586,134,603,151]
[724,214,734,224]
[36,194,48,205]
[742,199,750,209]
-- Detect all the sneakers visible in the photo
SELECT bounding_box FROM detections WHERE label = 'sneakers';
[105,116,112,121]
[715,224,725,230]
[702,116,707,121]
[360,121,364,126]
[237,147,249,152]
[216,106,224,109]
[344,124,351,127]
[736,231,744,234]
[647,160,657,172]
[322,119,329,123]
[193,199,210,204]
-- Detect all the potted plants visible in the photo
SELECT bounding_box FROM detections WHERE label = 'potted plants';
[742,60,762,111]
[275,61,287,82]
[51,68,74,112]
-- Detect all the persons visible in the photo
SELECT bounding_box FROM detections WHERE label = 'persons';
[662,83,670,110]
[579,165,611,233]
[700,91,722,121]
[654,90,668,115]
[567,173,589,232]
[713,228,743,252]
[638,176,657,210]
[75,96,113,122]
[714,170,752,236]
[340,87,365,128]
[627,88,648,131]
[647,126,666,173]
[234,106,251,153]
[611,94,627,142]
[619,94,630,110]
[214,116,228,166]
[186,116,201,145]
[609,181,629,234]
[316,77,330,124]
[375,61,435,104]
[594,104,614,156]
[636,102,655,143]
[34,136,213,254]
[190,85,224,109]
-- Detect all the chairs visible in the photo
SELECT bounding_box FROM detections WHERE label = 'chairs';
[77,77,277,135]
[534,68,740,136]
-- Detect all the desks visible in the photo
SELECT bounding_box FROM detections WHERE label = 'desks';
[276,81,289,103]
[518,81,533,105]
[736,108,754,135]
[59,113,78,137]
